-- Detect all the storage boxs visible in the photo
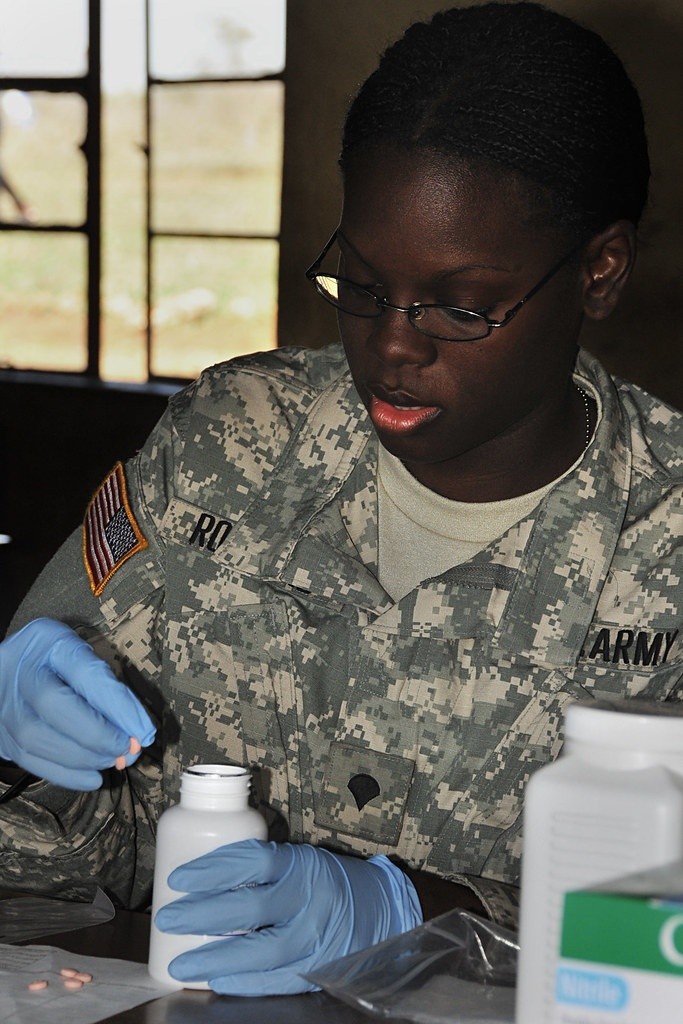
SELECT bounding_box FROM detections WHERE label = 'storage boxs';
[558,864,683,1024]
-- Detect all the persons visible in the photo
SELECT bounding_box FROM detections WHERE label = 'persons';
[1,0,683,995]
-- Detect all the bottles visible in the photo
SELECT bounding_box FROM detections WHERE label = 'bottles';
[515,697,683,1024]
[148,764,268,990]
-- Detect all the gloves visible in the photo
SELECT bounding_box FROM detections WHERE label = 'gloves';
[154,838,424,997]
[0,617,156,791]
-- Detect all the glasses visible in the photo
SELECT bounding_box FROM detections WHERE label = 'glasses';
[303,217,611,342]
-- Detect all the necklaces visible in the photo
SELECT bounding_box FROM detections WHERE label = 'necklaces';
[575,384,589,448]
